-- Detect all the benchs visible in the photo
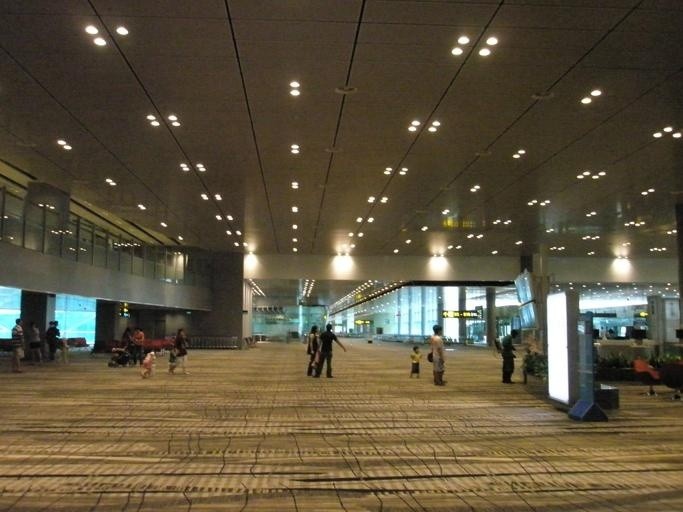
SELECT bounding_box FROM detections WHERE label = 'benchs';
[67,336,89,352]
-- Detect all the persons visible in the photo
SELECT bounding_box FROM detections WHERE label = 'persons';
[304,324,318,376]
[26,321,46,366]
[105,322,191,379]
[500,329,519,384]
[311,323,347,378]
[46,320,60,361]
[429,324,448,385]
[45,319,54,344]
[600,327,605,337]
[410,346,424,380]
[10,319,25,373]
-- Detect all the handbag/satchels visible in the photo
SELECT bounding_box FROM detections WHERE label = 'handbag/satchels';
[428,353,433,362]
[308,342,317,354]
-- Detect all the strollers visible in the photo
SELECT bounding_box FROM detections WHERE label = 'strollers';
[108,342,132,368]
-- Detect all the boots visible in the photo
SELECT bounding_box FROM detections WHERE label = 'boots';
[433,371,446,385]
[314,369,321,377]
[327,369,332,378]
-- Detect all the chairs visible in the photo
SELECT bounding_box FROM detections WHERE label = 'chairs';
[631,357,683,401]
